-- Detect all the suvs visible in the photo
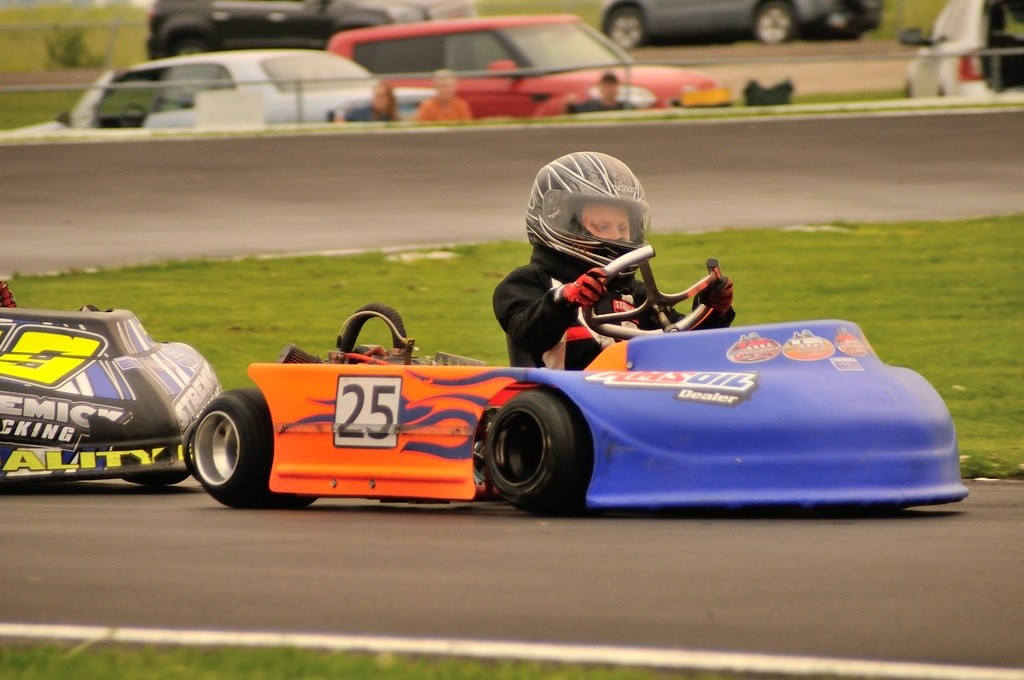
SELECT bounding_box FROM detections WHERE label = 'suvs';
[146,0,475,59]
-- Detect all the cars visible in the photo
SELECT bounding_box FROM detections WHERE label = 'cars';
[603,0,882,47]
[904,0,1024,112]
[0,52,439,138]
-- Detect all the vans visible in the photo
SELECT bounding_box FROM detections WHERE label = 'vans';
[327,14,724,116]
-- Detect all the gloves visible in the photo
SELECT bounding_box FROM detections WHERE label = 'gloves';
[698,276,734,315]
[554,267,609,308]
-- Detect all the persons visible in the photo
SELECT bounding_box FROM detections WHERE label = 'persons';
[491,151,736,370]
[414,69,473,121]
[568,73,640,114]
[334,80,402,123]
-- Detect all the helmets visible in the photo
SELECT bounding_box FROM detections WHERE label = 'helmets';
[526,152,649,278]
